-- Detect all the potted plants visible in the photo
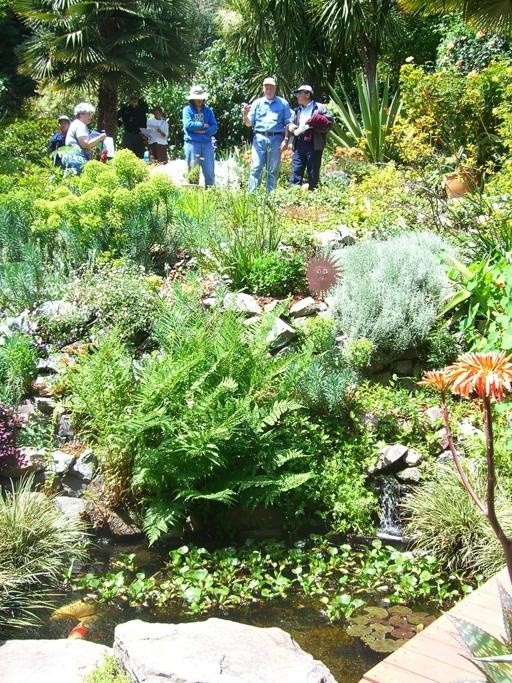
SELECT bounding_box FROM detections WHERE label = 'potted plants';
[440,145,481,201]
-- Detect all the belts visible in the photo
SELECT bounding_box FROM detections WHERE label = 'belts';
[256,131,282,136]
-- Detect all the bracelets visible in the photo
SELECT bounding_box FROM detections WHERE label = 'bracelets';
[284,137,289,140]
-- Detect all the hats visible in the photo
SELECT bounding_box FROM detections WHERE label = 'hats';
[185,86,208,100]
[293,85,314,95]
[263,78,275,85]
[59,116,69,121]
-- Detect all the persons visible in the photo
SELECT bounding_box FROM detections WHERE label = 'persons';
[182,86,218,189]
[64,101,107,164]
[144,106,169,164]
[50,115,71,148]
[241,77,291,193]
[287,85,333,188]
[116,93,146,159]
